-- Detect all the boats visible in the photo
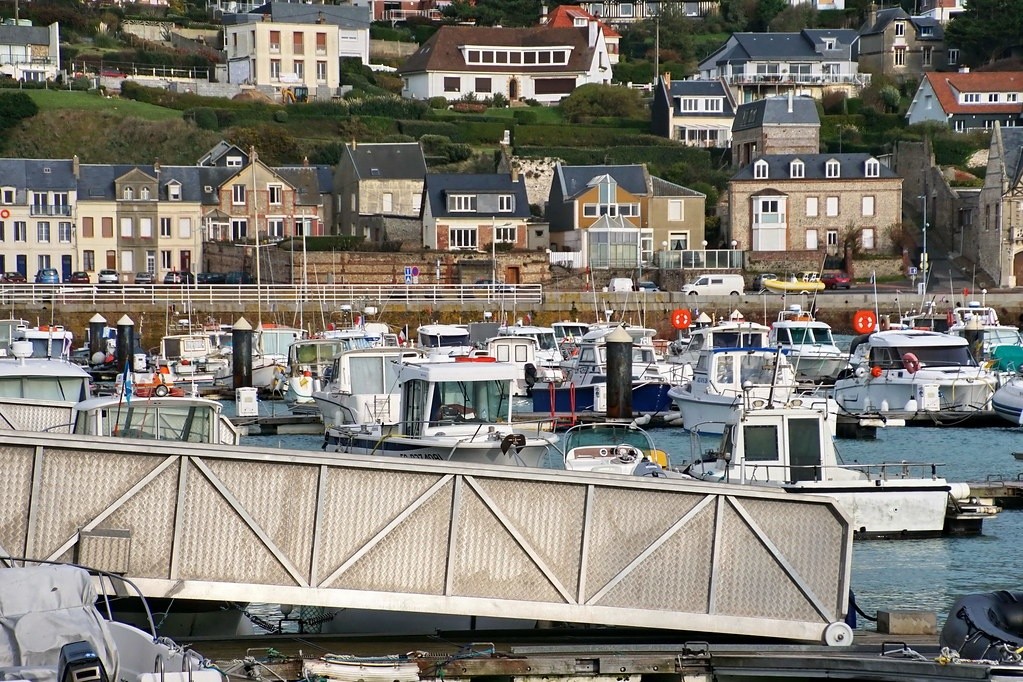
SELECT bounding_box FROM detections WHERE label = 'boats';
[936,581,1023,670]
[0,555,225,682]
[1,141,1023,541]
[763,278,826,292]
[302,653,427,682]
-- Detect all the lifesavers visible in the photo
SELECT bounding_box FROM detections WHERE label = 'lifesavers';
[478,357,497,362]
[902,353,922,374]
[455,357,478,362]
[39,325,57,331]
[879,314,890,331]
[947,310,953,326]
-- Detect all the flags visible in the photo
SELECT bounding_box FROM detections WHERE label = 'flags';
[397,324,408,344]
[123,357,134,405]
[355,315,366,326]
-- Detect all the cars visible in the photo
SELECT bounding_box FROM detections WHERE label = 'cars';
[198,272,226,284]
[98,269,120,284]
[0,271,27,284]
[34,268,60,284]
[134,271,155,284]
[164,272,207,285]
[224,271,255,284]
[473,279,518,292]
[631,282,661,292]
[68,271,91,284]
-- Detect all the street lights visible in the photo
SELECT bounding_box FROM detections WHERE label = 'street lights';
[917,195,927,294]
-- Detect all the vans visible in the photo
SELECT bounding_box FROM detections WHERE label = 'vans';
[607,277,634,292]
[680,273,746,296]
[796,270,820,282]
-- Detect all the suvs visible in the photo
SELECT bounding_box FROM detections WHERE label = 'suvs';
[820,272,852,290]
[752,273,778,292]
